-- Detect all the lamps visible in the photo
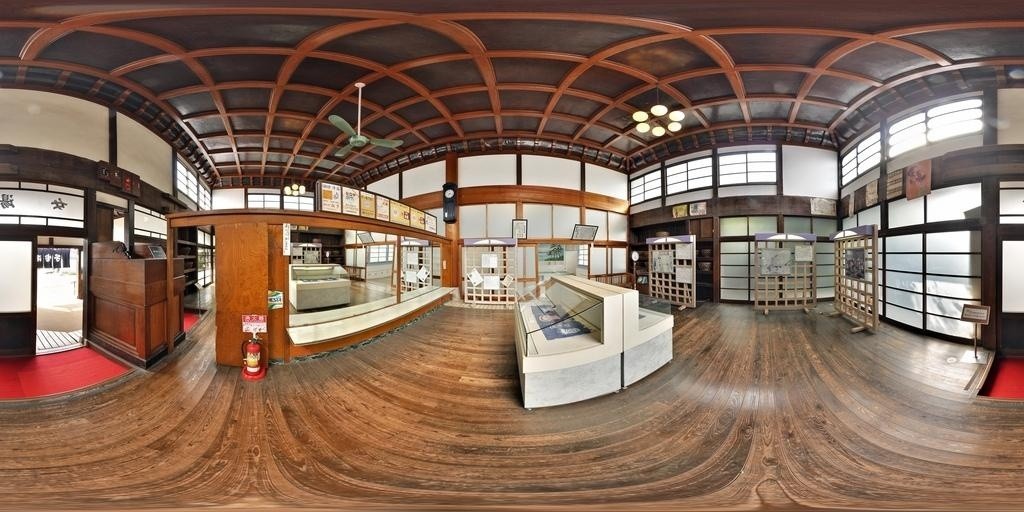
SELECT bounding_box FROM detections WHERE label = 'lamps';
[278,153,306,198]
[631,83,685,138]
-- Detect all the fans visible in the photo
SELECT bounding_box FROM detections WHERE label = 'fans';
[325,82,405,159]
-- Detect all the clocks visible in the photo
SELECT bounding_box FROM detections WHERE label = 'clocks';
[444,189,454,199]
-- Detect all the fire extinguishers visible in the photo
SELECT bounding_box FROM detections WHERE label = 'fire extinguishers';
[241,338,263,376]
